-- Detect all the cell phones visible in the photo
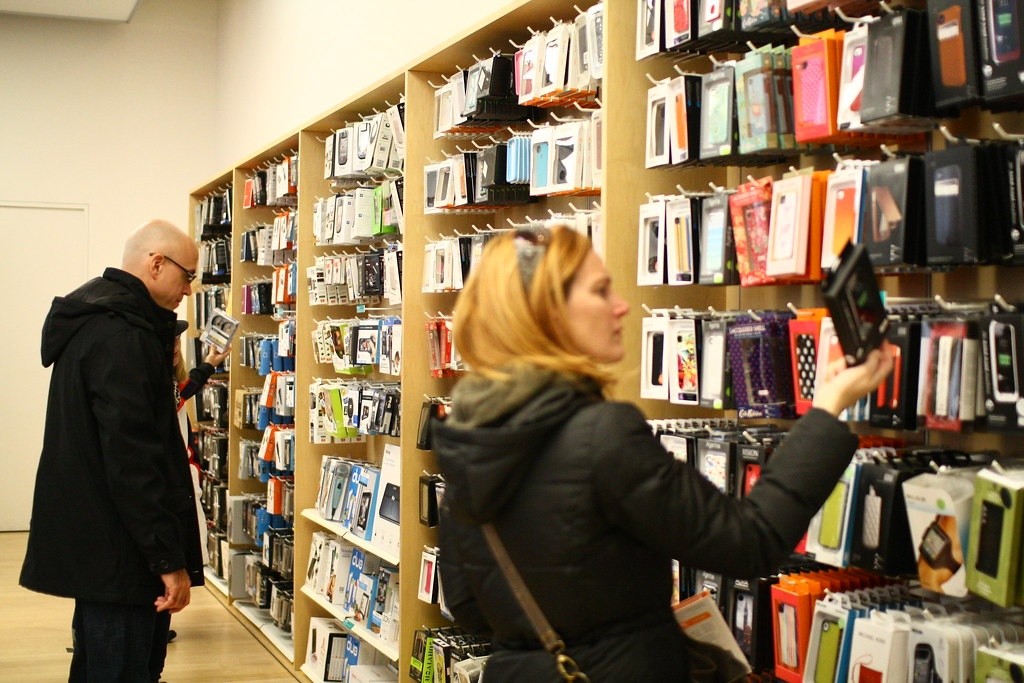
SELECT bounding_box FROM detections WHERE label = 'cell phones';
[338,132,347,165]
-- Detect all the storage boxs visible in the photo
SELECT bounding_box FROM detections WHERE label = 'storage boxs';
[372,443,401,557]
[354,572,378,630]
[333,191,360,247]
[334,127,355,177]
[353,467,381,541]
[785,47,808,152]
[323,539,354,604]
[363,113,393,173]
[305,531,334,594]
[343,547,382,612]
[373,186,395,237]
[199,308,240,354]
[861,156,926,269]
[734,44,779,154]
[901,473,975,598]
[926,142,996,266]
[861,9,919,126]
[850,460,917,578]
[772,45,795,151]
[352,186,376,240]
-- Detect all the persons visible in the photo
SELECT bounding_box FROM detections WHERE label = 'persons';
[430,223,894,683]
[18,219,232,683]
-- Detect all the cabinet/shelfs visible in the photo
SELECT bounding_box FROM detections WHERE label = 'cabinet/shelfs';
[187,0,1024,683]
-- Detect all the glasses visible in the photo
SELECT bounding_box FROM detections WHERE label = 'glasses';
[513,222,551,304]
[149,252,196,286]
